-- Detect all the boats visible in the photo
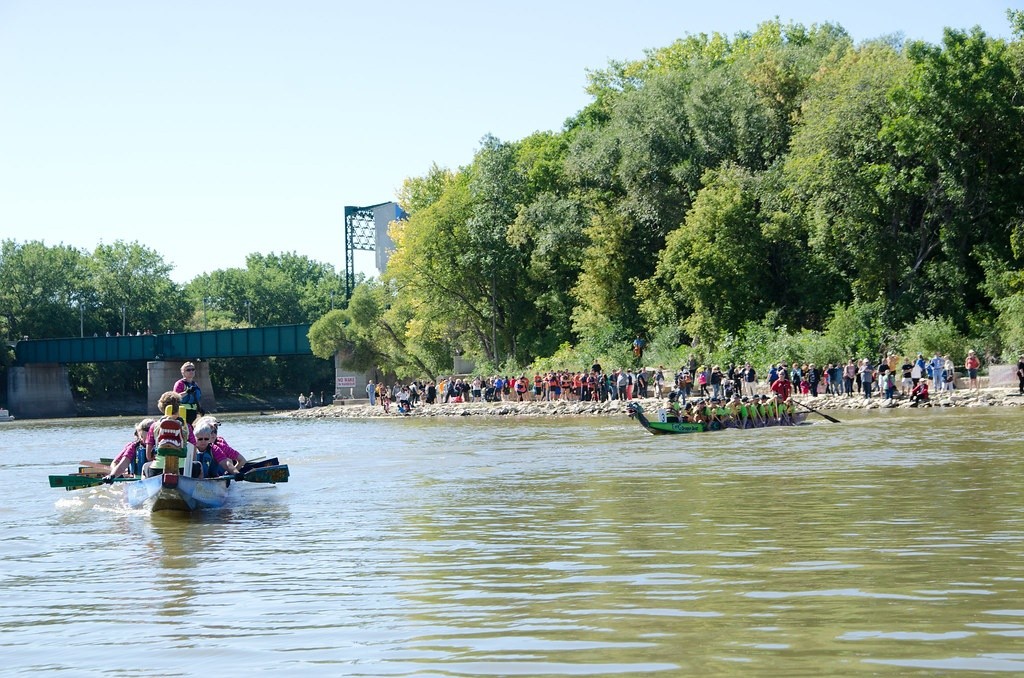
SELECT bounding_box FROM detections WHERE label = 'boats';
[117,472,250,516]
[626,399,811,436]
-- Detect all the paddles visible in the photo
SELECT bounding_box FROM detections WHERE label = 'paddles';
[699,396,795,429]
[792,400,840,423]
[48,456,290,492]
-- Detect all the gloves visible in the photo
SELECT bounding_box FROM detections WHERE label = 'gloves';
[186,386,196,395]
[197,407,205,417]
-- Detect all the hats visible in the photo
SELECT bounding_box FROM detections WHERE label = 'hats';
[919,378,927,382]
[969,350,976,354]
[668,392,793,408]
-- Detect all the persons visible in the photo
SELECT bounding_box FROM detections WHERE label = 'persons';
[93,329,175,335]
[102,361,246,479]
[297,336,1024,429]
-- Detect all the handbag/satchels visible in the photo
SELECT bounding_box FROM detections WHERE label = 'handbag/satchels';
[888,375,893,388]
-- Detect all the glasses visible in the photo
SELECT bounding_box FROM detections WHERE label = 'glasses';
[143,427,150,432]
[197,437,209,441]
[185,368,195,372]
